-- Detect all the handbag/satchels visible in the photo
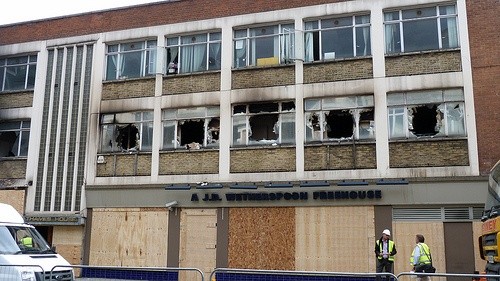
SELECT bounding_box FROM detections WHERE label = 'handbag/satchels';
[417,265,435,277]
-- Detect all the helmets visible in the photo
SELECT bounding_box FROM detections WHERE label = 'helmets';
[382,229,391,236]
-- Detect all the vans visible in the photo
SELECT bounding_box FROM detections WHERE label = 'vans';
[1,202,74,281]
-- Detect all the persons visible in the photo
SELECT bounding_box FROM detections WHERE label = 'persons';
[412,234,436,280]
[16,234,35,248]
[373,230,399,281]
[167,61,178,75]
